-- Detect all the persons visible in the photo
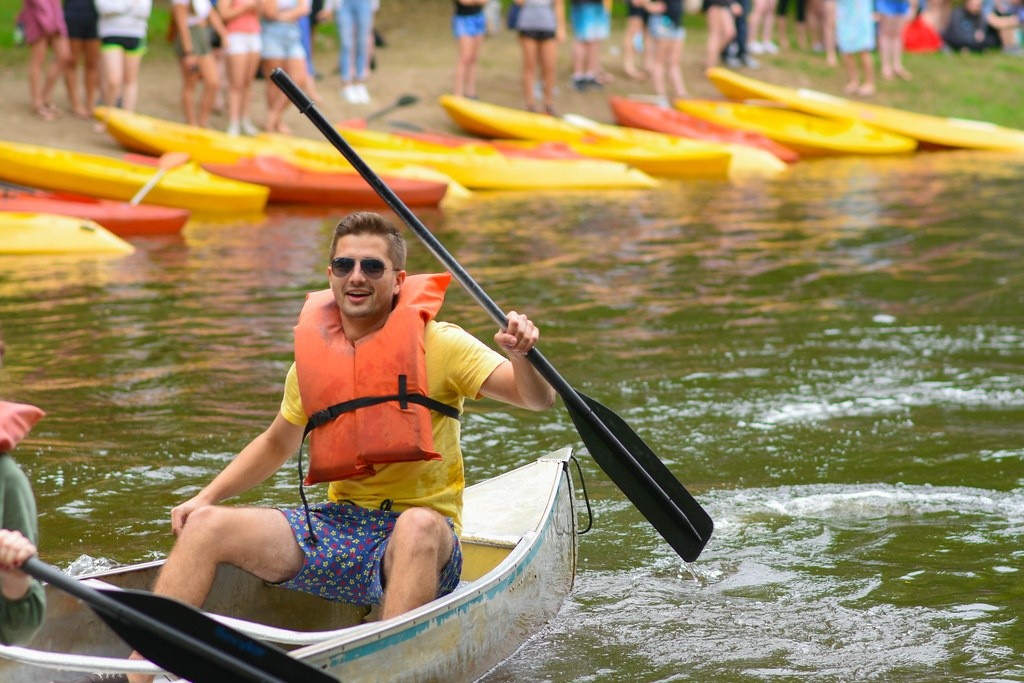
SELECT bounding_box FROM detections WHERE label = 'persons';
[13,0,99,122]
[0,341,47,648]
[50,211,557,683]
[513,0,567,120]
[571,0,1024,110]
[93,0,151,135]
[167,0,380,137]
[453,0,487,100]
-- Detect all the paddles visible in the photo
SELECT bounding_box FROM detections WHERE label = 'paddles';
[19,554,344,683]
[130,149,189,205]
[340,95,418,129]
[270,66,714,563]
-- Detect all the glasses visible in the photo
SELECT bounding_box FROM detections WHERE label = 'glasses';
[330,257,401,281]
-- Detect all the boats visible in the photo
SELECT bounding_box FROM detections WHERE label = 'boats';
[0,444,581,683]
[0,66,1024,259]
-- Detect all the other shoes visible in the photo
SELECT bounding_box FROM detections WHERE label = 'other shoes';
[28,102,63,122]
[227,119,257,135]
[343,83,371,104]
[747,40,778,55]
[575,78,602,93]
